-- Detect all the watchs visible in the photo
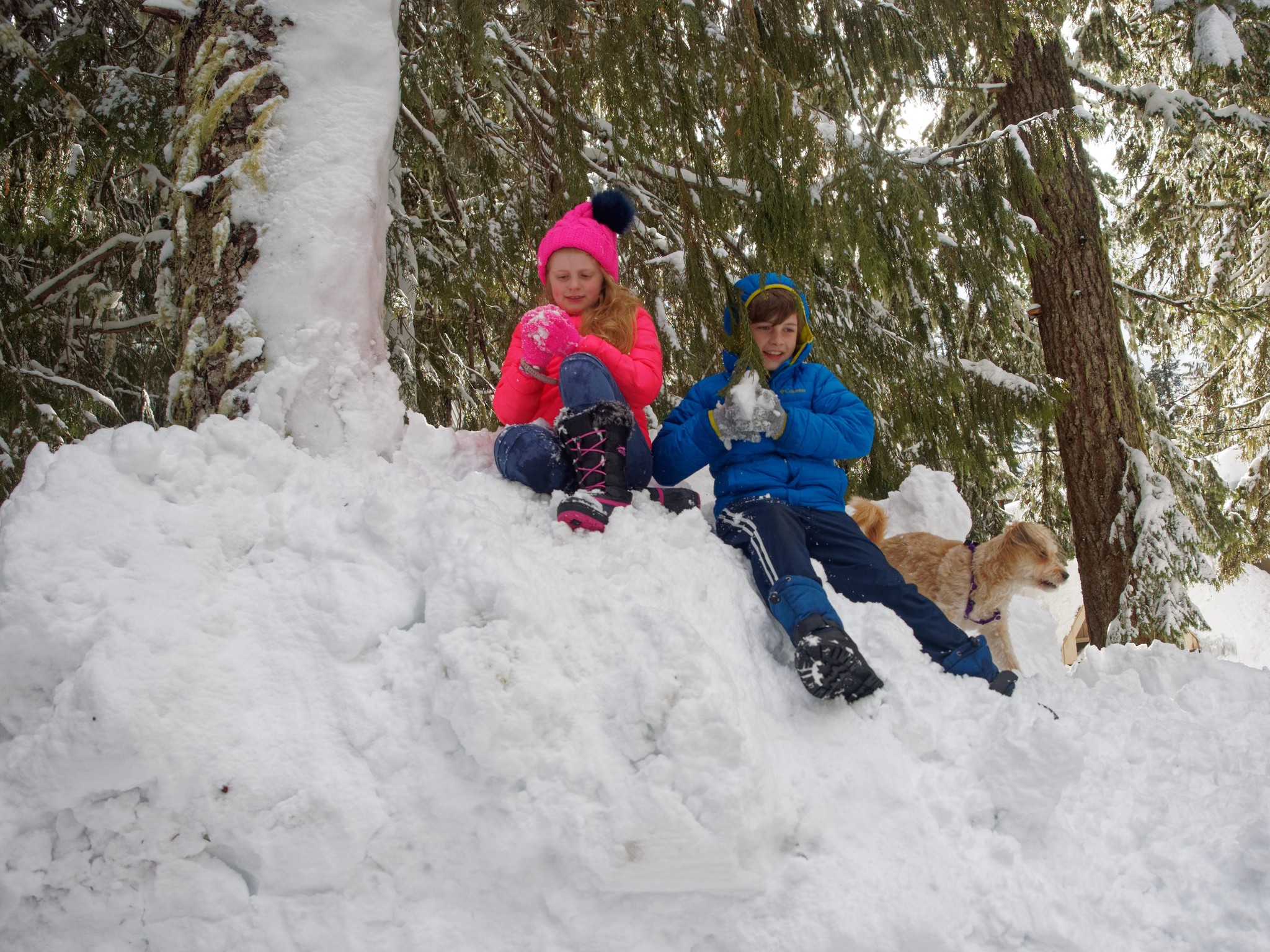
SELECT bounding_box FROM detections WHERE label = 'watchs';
[519,357,558,385]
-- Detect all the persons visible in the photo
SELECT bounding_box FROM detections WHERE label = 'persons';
[493,188,701,533]
[650,273,1019,707]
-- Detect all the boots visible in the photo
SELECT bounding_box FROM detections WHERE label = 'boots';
[769,576,883,703]
[945,635,1017,696]
[648,487,701,513]
[556,399,633,532]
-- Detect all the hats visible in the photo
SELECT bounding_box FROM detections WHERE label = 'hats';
[538,191,635,283]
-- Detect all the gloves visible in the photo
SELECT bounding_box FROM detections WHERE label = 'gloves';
[527,310,578,357]
[725,387,786,439]
[711,370,761,443]
[521,304,559,366]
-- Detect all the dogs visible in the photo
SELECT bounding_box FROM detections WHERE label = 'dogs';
[846,495,1069,671]
